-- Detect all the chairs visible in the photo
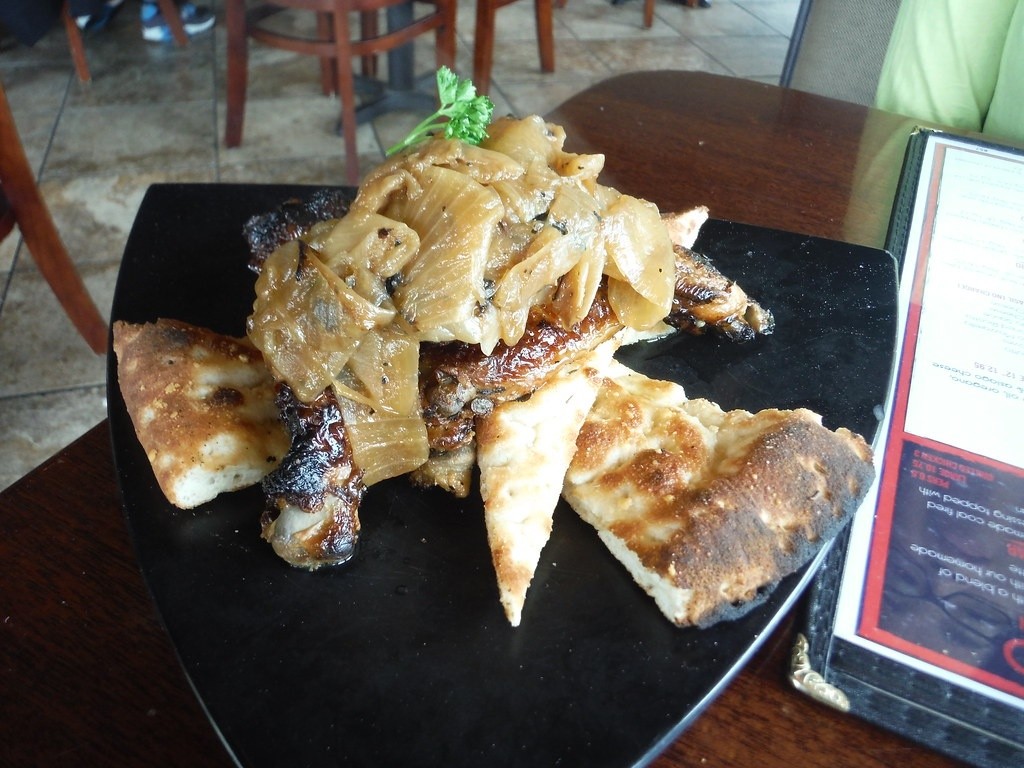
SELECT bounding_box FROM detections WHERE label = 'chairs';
[0,0,704,357]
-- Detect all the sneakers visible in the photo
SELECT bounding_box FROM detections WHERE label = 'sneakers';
[74,0,121,37]
[141,2,217,41]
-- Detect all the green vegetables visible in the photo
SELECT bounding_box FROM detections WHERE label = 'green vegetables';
[386,66,493,156]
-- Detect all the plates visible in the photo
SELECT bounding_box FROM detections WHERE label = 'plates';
[107,184,899,768]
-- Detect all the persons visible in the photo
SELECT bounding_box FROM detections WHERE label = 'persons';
[70,0,216,43]
[870,0,1024,155]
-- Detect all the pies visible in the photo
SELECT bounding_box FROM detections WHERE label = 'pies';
[116,317,302,508]
[559,352,879,630]
[483,204,712,628]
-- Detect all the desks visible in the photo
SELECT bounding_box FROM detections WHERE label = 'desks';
[0,69,1024,768]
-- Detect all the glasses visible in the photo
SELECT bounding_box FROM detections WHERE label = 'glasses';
[887,545,1019,645]
